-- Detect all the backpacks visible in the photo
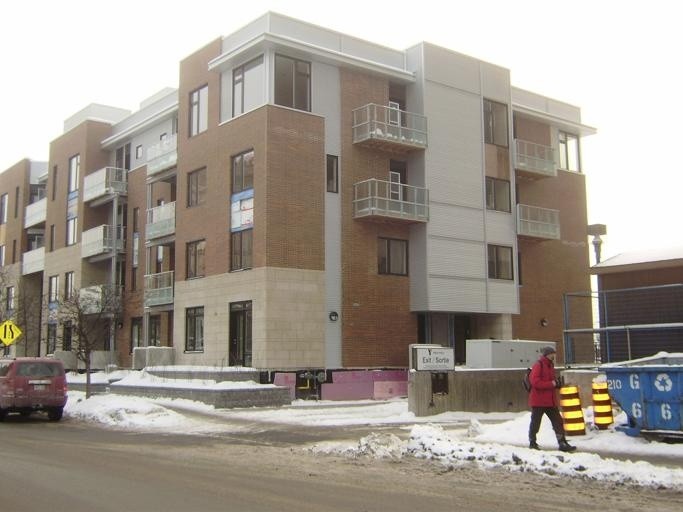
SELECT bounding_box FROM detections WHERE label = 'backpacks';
[522,360,544,393]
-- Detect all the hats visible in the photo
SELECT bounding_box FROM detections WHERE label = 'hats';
[543,346,556,356]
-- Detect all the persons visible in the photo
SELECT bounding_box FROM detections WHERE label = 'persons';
[527,346,576,452]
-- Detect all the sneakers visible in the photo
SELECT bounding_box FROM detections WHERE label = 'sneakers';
[559,440,576,452]
[529,441,541,450]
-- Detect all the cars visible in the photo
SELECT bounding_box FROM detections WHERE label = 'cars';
[0,356,68,422]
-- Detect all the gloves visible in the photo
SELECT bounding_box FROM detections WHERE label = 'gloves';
[554,376,562,388]
